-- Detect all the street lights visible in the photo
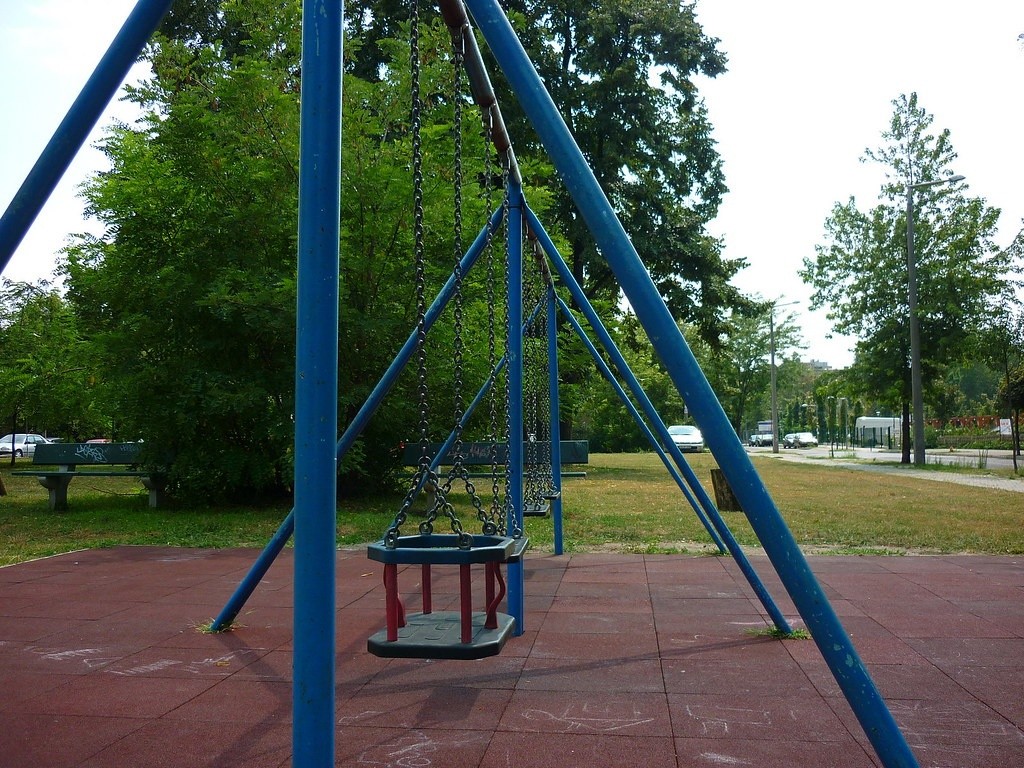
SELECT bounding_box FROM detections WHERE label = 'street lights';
[839,398,848,450]
[770,299,802,454]
[801,403,819,442]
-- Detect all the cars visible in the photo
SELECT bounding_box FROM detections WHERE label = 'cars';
[793,432,818,449]
[663,425,703,453]
[0,434,53,459]
[86,439,113,443]
[748,434,774,447]
[45,437,64,443]
[783,434,796,449]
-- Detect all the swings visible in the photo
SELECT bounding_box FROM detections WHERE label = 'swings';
[475,97,562,564]
[365,1,517,664]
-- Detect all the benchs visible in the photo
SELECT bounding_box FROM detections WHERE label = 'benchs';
[400,439,589,485]
[11,441,173,510]
